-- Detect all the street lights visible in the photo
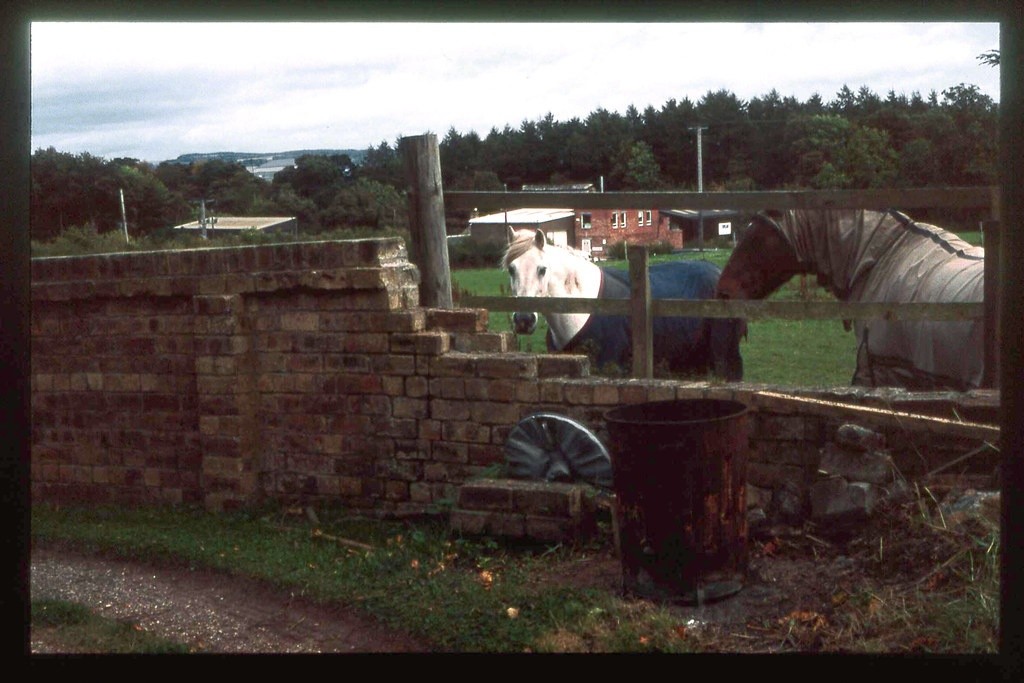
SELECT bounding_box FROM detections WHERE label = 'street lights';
[689,125,708,192]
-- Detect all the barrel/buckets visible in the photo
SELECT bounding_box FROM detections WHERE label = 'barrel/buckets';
[603,399,755,604]
